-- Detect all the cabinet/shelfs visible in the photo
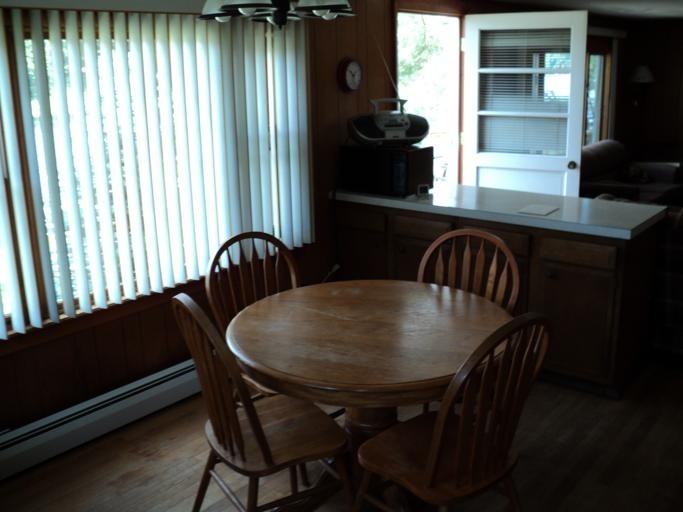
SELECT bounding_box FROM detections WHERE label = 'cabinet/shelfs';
[328,200,657,399]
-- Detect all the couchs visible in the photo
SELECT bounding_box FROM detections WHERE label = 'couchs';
[578,138,682,204]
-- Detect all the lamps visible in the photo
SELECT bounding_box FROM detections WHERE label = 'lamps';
[197,0,359,27]
[630,64,656,85]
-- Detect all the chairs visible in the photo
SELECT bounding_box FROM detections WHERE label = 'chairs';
[171,292,352,511]
[417,228,520,413]
[206,231,343,488]
[358,310,550,512]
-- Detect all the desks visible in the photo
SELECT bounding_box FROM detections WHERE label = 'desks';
[226,280,514,510]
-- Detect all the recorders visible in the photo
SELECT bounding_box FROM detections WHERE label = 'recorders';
[347,97,431,144]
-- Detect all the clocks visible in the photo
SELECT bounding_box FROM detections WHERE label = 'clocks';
[336,55,363,94]
